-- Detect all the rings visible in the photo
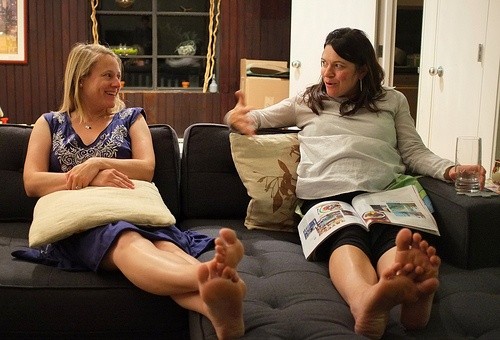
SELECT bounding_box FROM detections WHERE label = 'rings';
[76,186,82,189]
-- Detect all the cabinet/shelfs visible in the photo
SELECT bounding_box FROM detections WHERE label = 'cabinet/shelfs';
[90,0,221,91]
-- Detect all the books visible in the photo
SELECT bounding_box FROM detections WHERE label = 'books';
[298,186,440,260]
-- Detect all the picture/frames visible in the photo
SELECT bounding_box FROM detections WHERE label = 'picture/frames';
[0,0,30,66]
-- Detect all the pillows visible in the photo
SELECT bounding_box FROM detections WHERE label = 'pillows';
[28,179,177,250]
[229,133,307,233]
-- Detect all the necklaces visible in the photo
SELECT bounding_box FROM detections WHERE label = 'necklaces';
[79,117,104,129]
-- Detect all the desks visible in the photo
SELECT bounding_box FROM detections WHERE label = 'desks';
[240,59,290,113]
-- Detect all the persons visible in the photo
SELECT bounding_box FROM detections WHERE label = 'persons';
[226,28,486,339]
[23,45,246,340]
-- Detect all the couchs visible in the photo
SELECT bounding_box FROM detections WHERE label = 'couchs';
[0,122,500,340]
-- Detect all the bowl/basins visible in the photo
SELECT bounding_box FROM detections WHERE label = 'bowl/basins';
[109,46,137,55]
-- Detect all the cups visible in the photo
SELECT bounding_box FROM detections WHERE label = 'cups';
[454,136,483,193]
[1,117,8,124]
[182,81,190,88]
